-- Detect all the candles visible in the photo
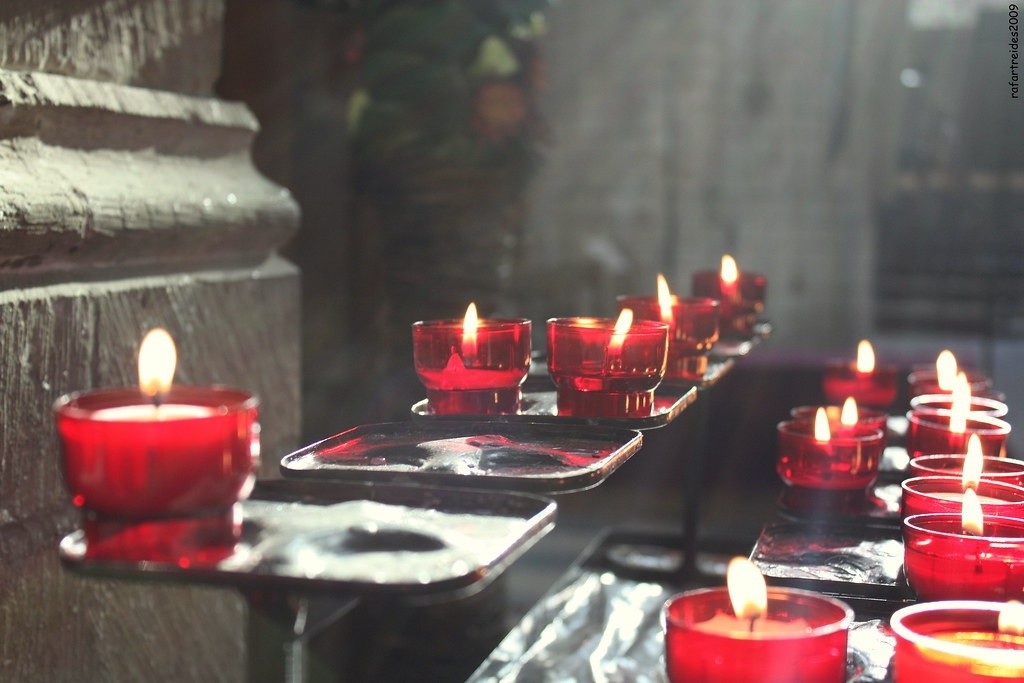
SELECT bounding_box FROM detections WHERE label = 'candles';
[823,340,898,410]
[776,406,884,515]
[890,600,1024,683]
[904,488,1024,602]
[547,308,669,418]
[905,385,1013,457]
[692,255,768,338]
[910,373,1008,420]
[791,397,886,455]
[618,273,720,380]
[412,302,532,416]
[664,556,853,683]
[53,328,262,566]
[908,350,991,402]
[909,433,1024,487]
[901,452,1024,519]
